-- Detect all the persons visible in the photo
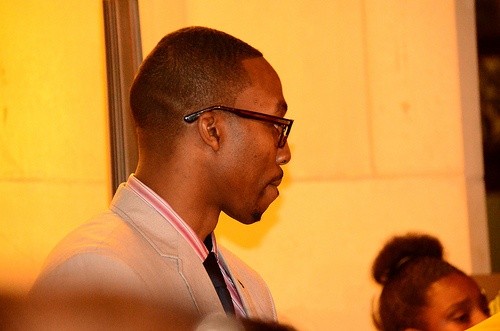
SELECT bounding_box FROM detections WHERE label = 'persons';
[31,26,294,322]
[373,235,492,331]
[0,289,298,331]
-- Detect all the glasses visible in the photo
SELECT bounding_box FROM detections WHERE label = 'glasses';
[184,106,294,149]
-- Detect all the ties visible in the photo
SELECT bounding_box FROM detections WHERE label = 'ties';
[202,252,238,321]
